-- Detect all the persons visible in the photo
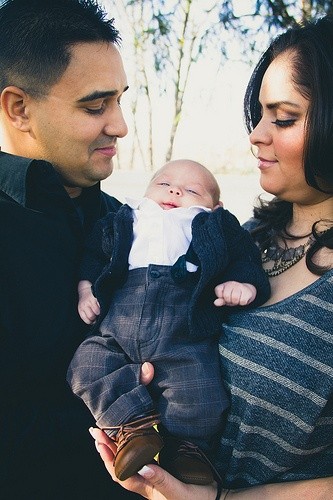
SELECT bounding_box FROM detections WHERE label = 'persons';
[89,16,333,500]
[0,0,128,500]
[67,159,272,485]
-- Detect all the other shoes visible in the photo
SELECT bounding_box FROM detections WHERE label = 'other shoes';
[99,414,165,481]
[158,440,221,485]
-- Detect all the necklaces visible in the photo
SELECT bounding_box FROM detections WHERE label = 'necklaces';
[255,231,325,277]
